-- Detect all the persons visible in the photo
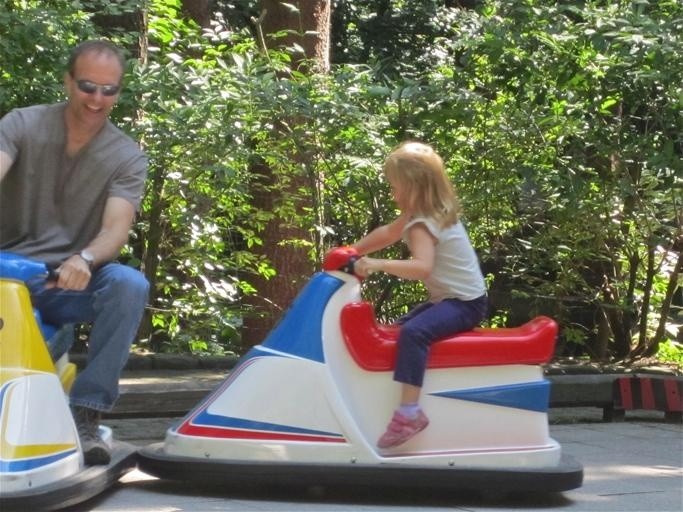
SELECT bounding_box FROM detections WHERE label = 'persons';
[350,137,492,448]
[0,39,151,466]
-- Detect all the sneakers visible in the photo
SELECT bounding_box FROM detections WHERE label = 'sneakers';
[377,406,430,449]
[68,405,111,466]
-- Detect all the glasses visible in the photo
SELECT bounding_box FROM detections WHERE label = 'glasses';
[72,76,121,96]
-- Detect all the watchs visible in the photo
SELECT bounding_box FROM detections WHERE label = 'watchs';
[74,247,97,273]
